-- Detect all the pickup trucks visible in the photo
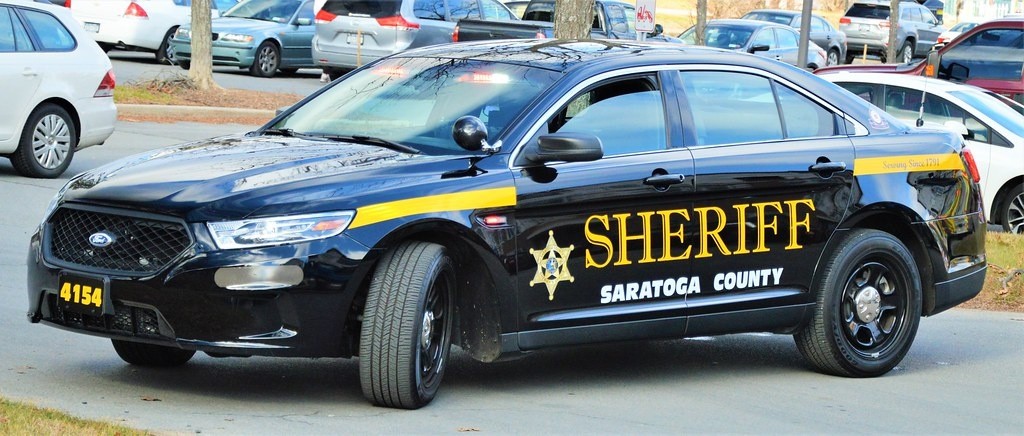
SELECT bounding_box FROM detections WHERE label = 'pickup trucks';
[454,0,663,43]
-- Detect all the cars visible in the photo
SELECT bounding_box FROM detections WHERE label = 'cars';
[742,11,847,68]
[166,0,322,76]
[34,1,245,57]
[678,19,827,72]
[895,0,944,24]
[811,72,1024,235]
[937,21,984,52]
[30,37,987,413]
[0,1,118,178]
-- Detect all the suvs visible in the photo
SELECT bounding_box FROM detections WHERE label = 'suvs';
[813,17,1024,110]
[312,0,523,82]
[839,1,948,66]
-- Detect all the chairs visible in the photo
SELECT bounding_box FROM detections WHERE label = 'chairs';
[448,76,663,164]
